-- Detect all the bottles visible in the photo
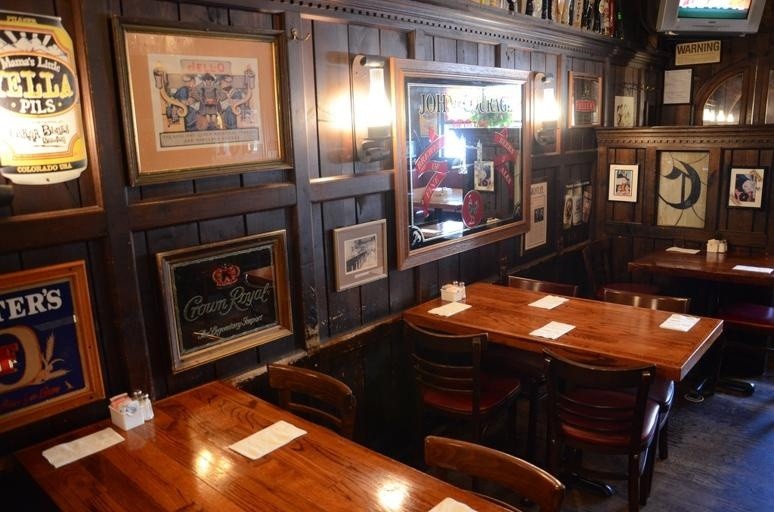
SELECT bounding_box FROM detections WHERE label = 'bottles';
[132,388,154,420]
[452,281,465,298]
[719,239,727,253]
[562,182,593,228]
[473,0,625,40]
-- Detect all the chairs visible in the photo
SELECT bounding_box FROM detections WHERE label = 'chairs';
[581,233,774,396]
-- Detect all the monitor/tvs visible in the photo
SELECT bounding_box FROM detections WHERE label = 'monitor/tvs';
[652,0,767,38]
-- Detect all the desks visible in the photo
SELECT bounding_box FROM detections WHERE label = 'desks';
[398,279,723,496]
[11,378,516,509]
[412,186,463,224]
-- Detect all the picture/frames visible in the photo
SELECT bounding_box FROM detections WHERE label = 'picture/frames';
[568,71,602,129]
[419,104,437,137]
[607,163,640,203]
[724,166,768,210]
[473,160,494,192]
[520,176,551,256]
[154,229,294,375]
[0,259,106,435]
[332,218,387,293]
[109,14,296,189]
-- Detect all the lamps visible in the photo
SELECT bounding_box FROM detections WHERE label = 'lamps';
[532,71,562,147]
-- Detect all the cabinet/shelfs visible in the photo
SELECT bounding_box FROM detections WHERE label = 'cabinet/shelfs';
[448,127,520,222]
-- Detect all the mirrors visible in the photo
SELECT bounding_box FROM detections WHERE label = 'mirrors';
[385,57,532,272]
